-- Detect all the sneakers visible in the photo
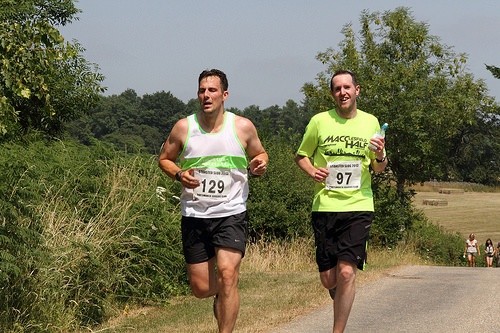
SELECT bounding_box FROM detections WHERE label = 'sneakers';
[329,287,337,300]
[213,293,218,320]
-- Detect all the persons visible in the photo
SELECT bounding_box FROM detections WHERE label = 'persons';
[463,233,480,267]
[295,71,388,332]
[158,70,269,333]
[484,238,500,267]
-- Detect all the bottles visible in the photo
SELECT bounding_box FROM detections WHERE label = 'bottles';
[365,122,389,160]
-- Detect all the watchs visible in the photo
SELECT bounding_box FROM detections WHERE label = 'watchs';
[175,170,182,181]
[375,155,385,163]
